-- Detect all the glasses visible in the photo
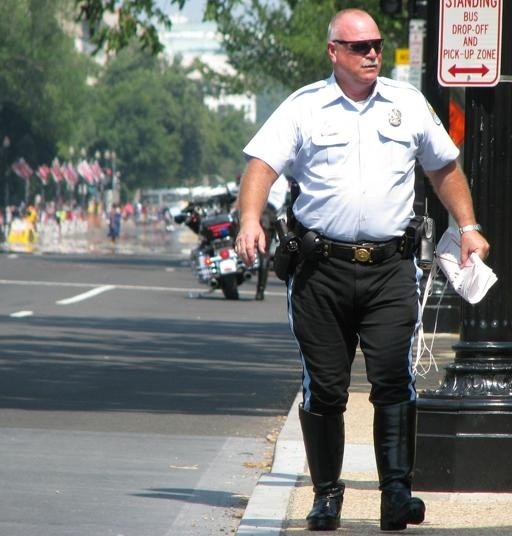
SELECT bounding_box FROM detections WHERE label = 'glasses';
[333,39,384,54]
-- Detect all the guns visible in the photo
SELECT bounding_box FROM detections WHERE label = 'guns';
[273,219,302,281]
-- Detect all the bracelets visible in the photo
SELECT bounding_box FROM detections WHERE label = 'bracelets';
[457,222,484,233]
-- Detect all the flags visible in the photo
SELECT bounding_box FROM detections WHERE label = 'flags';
[9,157,109,187]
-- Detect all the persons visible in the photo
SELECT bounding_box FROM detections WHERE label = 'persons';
[253,173,291,300]
[104,197,172,247]
[234,8,493,530]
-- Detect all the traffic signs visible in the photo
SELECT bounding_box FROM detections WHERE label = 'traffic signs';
[438,0,503,88]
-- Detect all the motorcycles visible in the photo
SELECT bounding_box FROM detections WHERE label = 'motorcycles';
[174,194,260,299]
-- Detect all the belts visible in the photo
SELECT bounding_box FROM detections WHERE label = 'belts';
[284,208,402,263]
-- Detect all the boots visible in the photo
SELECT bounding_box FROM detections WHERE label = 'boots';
[298,402,345,531]
[373,399,424,530]
